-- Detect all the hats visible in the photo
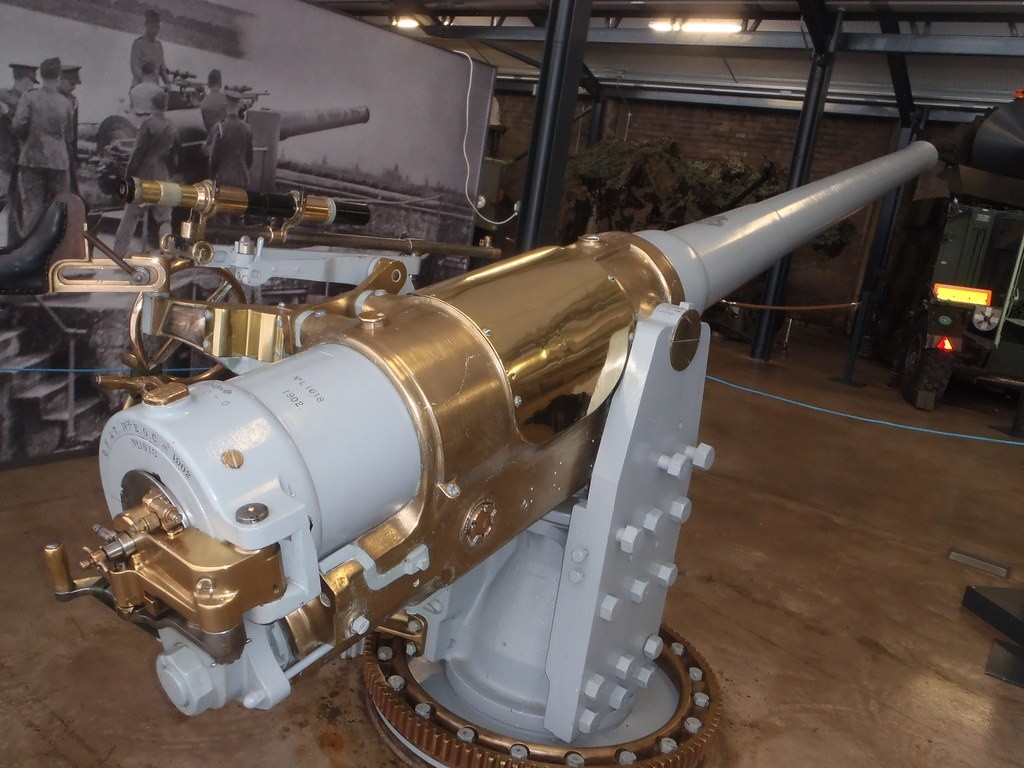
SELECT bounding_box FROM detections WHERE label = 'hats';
[60,64,82,86]
[224,90,243,103]
[10,61,41,83]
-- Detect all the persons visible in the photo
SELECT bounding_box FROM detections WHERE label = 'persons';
[1,9,254,250]
